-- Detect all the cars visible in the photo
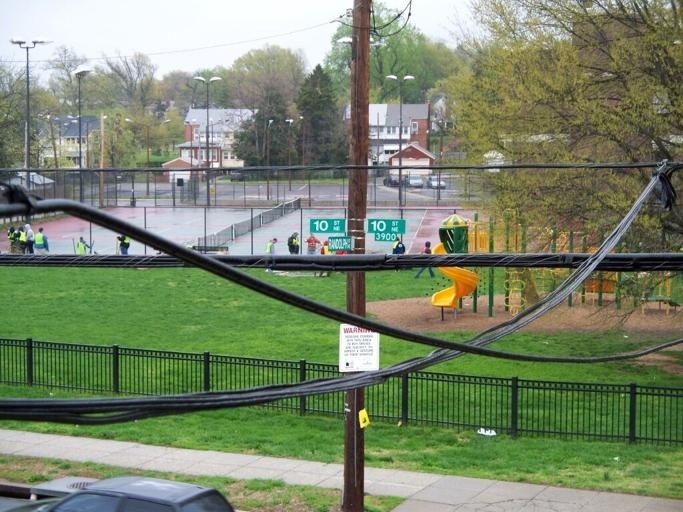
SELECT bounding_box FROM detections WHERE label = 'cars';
[379,170,448,190]
[0,471,237,512]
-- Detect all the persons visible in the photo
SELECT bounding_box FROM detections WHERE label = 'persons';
[392,238,405,254]
[117,235,129,255]
[266,239,277,273]
[288,232,299,254]
[8,224,49,255]
[415,241,435,279]
[76,237,90,255]
[318,241,333,277]
[304,234,322,254]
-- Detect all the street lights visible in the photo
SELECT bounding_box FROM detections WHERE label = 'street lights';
[338,35,387,52]
[73,61,93,201]
[384,73,415,205]
[95,110,308,209]
[194,74,226,206]
[6,37,56,232]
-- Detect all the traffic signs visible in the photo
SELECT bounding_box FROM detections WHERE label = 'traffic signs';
[328,236,352,252]
[367,218,407,235]
[373,232,397,241]
[308,217,346,235]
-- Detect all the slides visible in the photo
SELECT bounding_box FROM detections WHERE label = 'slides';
[431,243,478,308]
